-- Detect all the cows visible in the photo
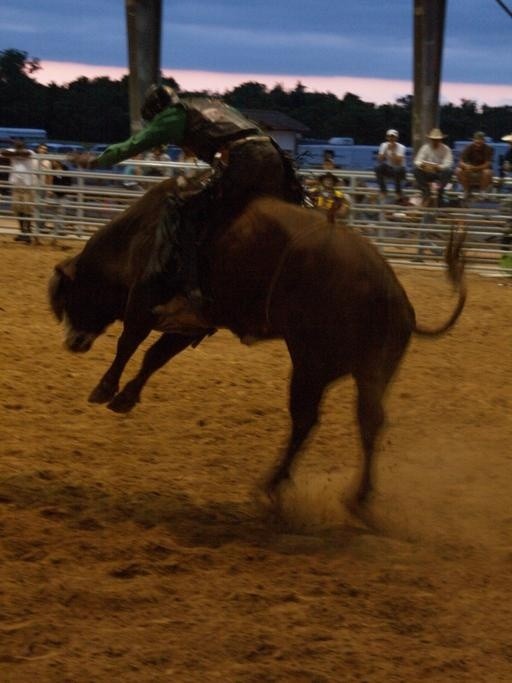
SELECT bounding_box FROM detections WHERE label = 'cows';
[45,170,470,520]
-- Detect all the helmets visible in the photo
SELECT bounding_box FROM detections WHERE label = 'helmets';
[140,83,180,121]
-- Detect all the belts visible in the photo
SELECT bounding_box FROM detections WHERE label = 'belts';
[233,136,270,146]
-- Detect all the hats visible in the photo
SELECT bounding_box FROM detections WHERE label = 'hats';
[319,172,338,183]
[386,129,399,137]
[426,128,448,140]
[501,134,512,142]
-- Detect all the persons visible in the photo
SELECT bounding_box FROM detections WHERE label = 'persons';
[411,130,453,208]
[385,155,511,263]
[456,131,495,203]
[303,148,380,237]
[79,142,198,218]
[78,83,307,302]
[0,135,76,249]
[374,130,408,202]
